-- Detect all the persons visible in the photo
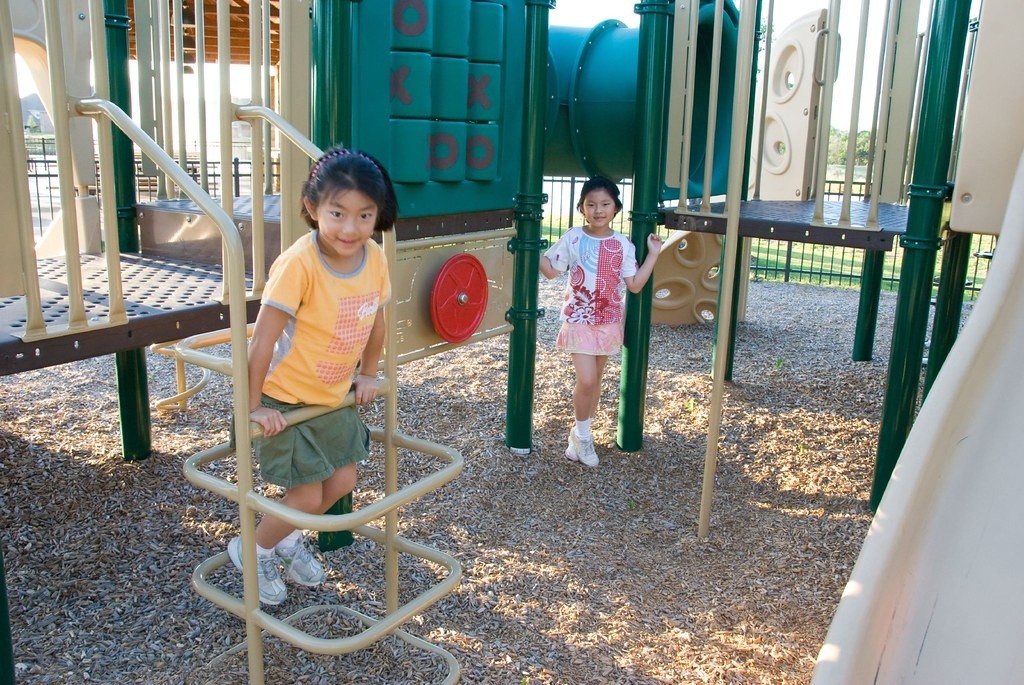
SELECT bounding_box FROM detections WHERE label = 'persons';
[540,177,662,466]
[227,150,397,605]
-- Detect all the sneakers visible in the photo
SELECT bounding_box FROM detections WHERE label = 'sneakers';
[227,529,324,606]
[565,427,600,466]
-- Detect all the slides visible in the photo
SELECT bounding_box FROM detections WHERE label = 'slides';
[805,147,1024,685]
